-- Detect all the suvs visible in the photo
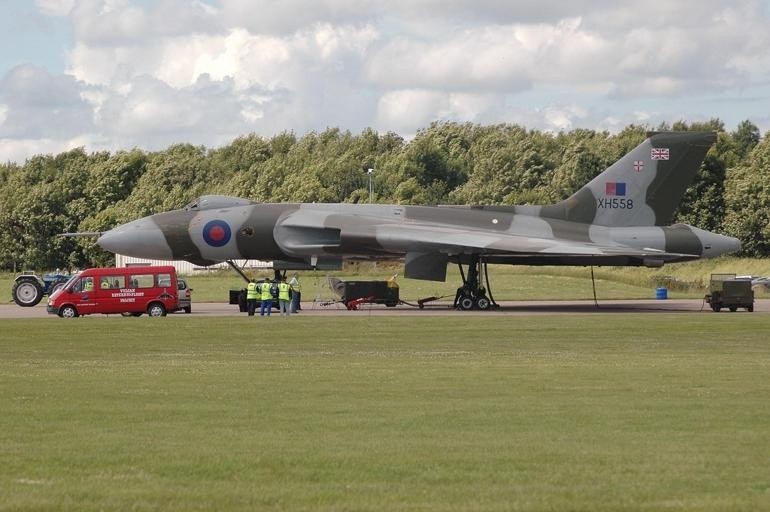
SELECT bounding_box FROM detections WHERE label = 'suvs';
[158,279,194,314]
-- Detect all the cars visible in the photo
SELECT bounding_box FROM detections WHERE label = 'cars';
[727,273,770,290]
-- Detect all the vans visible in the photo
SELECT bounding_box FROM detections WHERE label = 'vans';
[46,265,179,319]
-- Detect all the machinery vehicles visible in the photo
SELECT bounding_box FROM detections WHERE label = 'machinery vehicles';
[11,267,80,307]
[703,272,756,314]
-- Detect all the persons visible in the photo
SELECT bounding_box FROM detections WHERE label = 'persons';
[100,277,111,289]
[295,278,304,310]
[82,278,93,291]
[259,277,274,316]
[245,277,260,316]
[276,276,293,317]
[289,271,301,314]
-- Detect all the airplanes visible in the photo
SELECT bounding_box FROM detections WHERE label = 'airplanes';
[89,132,751,314]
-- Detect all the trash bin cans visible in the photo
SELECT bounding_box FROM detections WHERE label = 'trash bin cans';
[657,288,666,299]
